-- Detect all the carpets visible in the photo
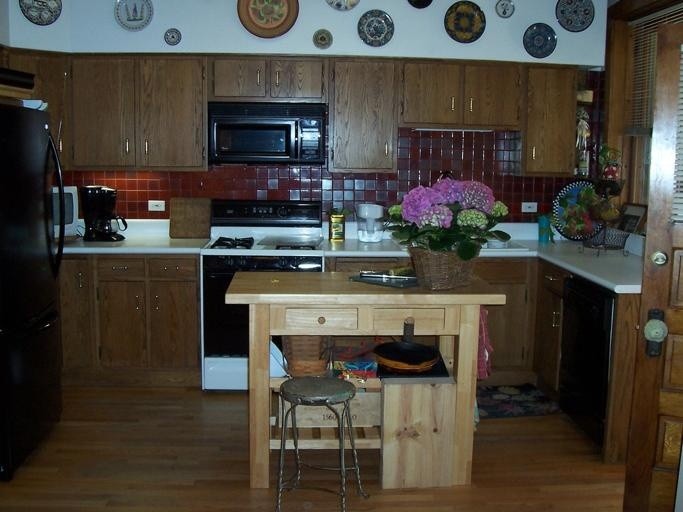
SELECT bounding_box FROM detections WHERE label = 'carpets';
[475,383,562,421]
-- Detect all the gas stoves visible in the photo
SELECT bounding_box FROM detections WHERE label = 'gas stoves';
[202,228,324,276]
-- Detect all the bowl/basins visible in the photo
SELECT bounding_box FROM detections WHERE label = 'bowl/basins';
[371,341,440,373]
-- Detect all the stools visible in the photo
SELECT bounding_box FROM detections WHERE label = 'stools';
[276,376,371,512]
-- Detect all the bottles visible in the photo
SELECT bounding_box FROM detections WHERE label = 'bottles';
[356,203,384,243]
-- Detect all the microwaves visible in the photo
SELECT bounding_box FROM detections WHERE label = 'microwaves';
[206,101,325,165]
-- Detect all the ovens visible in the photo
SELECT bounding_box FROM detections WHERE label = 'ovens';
[201,276,283,356]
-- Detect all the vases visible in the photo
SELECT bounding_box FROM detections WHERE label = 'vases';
[408,245,473,290]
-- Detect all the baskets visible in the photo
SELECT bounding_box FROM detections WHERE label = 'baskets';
[407,245,484,291]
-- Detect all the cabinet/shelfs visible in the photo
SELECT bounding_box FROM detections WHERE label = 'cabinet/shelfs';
[225,271,508,512]
[59,252,202,389]
[334,258,565,391]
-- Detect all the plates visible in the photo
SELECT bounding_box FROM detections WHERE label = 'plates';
[113,0,153,32]
[325,0,361,11]
[443,1,595,58]
[407,1,433,9]
[18,0,62,26]
[163,28,181,46]
[236,1,299,39]
[357,10,394,48]
[312,30,333,49]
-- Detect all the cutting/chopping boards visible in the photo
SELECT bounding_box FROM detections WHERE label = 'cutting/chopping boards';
[168,197,211,239]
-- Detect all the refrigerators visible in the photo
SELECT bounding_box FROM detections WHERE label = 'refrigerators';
[0,103,63,483]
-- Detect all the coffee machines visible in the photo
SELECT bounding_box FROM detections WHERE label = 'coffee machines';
[77,185,126,242]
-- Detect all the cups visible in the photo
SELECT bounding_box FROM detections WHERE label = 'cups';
[538,217,550,242]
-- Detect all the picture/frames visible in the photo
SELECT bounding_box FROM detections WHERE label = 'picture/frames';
[617,201,648,233]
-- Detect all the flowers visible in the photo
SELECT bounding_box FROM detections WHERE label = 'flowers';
[375,176,510,261]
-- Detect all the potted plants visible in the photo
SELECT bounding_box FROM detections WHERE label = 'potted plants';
[598,145,623,179]
